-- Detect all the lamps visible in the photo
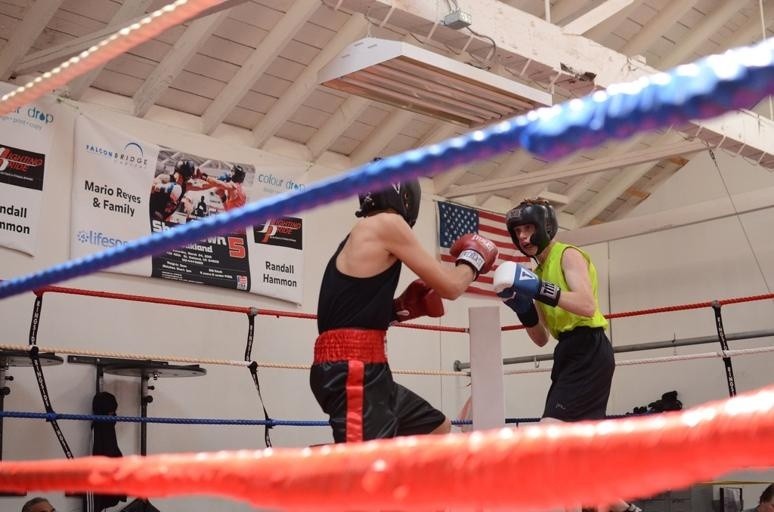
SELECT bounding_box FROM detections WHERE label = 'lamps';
[316,20,553,131]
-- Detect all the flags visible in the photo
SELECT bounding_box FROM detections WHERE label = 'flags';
[435,200,532,297]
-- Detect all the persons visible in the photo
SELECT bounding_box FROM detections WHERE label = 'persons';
[192,166,246,209]
[181,192,221,215]
[20,496,56,512]
[746,484,774,511]
[308,156,499,444]
[493,196,642,512]
[151,161,232,220]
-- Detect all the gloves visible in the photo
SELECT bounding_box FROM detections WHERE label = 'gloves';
[450,234,498,282]
[495,261,562,308]
[217,172,228,180]
[159,182,182,202]
[392,278,446,322]
[215,187,225,198]
[494,277,539,328]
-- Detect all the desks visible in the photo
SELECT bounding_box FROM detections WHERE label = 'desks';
[0,347,206,512]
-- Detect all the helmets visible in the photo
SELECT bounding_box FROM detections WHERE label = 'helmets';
[174,160,195,177]
[506,198,559,257]
[230,166,246,182]
[356,155,421,229]
